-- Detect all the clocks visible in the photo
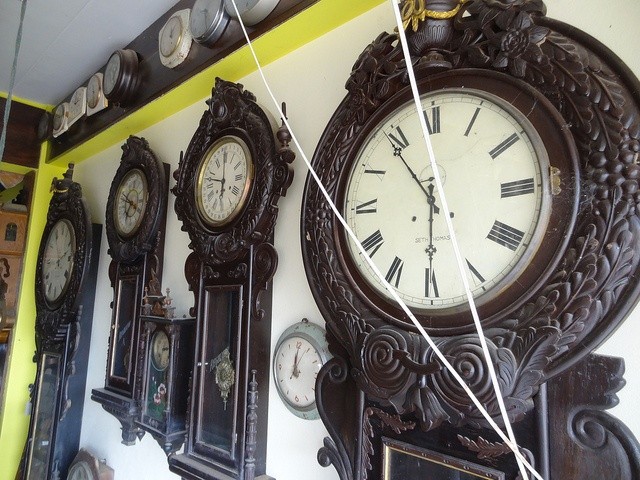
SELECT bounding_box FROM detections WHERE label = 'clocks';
[52,102,69,138]
[69,87,87,127]
[170,78,292,480]
[301,1,639,479]
[158,8,190,70]
[101,48,138,103]
[190,0,231,48]
[134,287,197,455]
[37,111,52,143]
[87,72,111,119]
[14,161,102,480]
[272,318,337,421]
[91,134,169,445]
[65,446,117,480]
[222,1,281,26]
